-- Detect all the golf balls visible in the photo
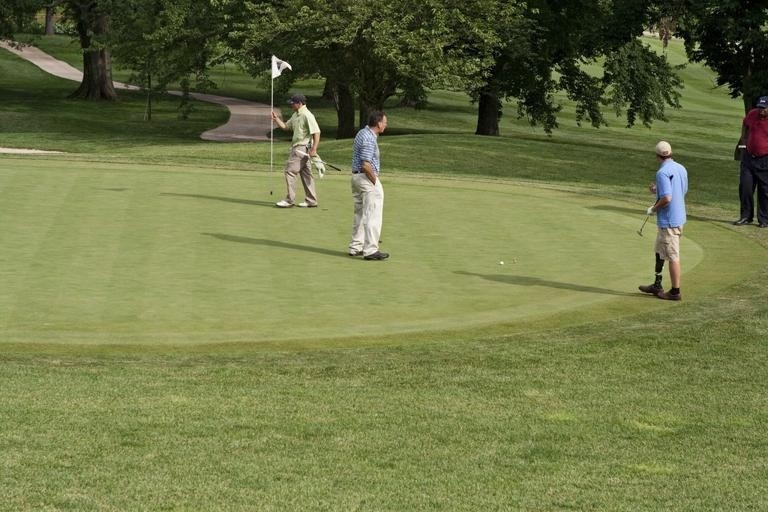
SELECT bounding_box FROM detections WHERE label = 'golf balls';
[500,261,504,264]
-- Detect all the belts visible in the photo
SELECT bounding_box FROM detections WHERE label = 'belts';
[746,153,765,159]
[353,170,363,174]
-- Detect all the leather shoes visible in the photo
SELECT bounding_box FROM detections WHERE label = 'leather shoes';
[759,223,767,227]
[734,218,754,226]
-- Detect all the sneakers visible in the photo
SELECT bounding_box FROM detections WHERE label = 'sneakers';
[276,200,295,208]
[365,251,389,261]
[349,251,363,256]
[639,285,664,295]
[298,201,317,207]
[657,291,681,300]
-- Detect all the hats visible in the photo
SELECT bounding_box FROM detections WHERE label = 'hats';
[756,96,768,108]
[284,94,307,105]
[655,141,672,157]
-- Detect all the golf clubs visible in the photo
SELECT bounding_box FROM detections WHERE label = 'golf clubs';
[298,151,340,171]
[637,175,673,237]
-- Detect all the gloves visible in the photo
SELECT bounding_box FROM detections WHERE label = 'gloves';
[647,206,656,216]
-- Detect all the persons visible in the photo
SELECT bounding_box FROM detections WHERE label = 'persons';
[272,94,321,208]
[732,96,768,229]
[638,140,689,301]
[348,111,390,261]
